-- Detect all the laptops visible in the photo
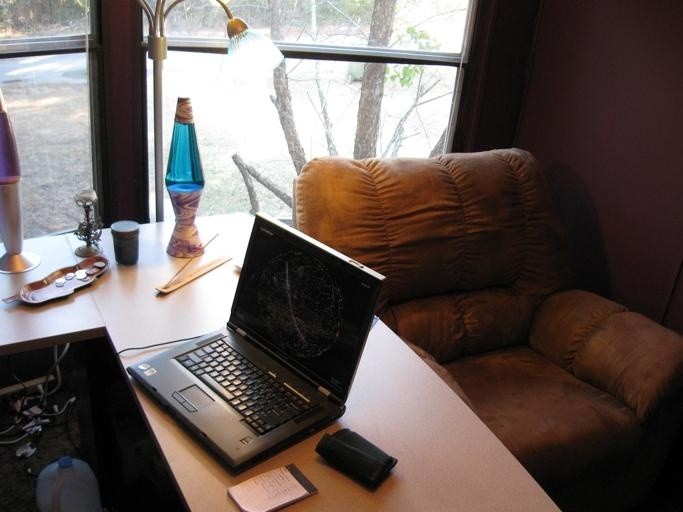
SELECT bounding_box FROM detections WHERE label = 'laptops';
[124,210,388,476]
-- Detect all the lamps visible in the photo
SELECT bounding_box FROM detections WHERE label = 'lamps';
[136,0,284,221]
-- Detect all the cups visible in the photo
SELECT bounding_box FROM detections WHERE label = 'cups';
[111,221,139,264]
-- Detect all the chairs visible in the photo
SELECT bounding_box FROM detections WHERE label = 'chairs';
[294,146,682,512]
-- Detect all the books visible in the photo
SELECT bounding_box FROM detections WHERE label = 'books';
[227,464,318,512]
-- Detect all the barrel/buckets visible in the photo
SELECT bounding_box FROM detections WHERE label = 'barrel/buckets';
[35,455,102,512]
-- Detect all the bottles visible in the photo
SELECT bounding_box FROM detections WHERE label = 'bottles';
[163,95,206,259]
[0,89,42,275]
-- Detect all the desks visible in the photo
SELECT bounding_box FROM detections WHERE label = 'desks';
[0,210,560,512]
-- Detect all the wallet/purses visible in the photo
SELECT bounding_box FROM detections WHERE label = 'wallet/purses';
[314,427,395,491]
